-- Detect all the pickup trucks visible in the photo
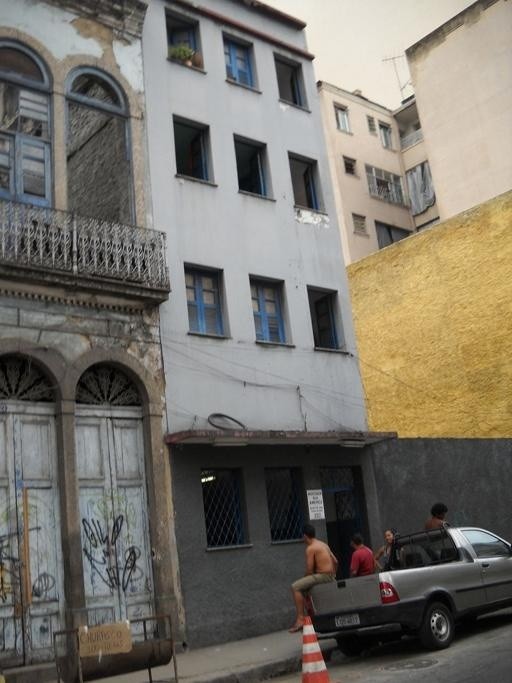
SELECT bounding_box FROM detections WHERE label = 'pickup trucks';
[299,515,512,651]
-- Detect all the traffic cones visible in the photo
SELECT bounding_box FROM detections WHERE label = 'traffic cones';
[295,610,334,682]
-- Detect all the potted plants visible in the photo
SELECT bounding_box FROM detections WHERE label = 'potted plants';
[167,45,195,67]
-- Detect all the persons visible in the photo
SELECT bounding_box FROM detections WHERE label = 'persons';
[426,501,447,529]
[373,527,404,572]
[289,522,338,633]
[349,533,375,578]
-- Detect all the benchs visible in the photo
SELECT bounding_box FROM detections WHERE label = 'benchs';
[53,614,180,683]
